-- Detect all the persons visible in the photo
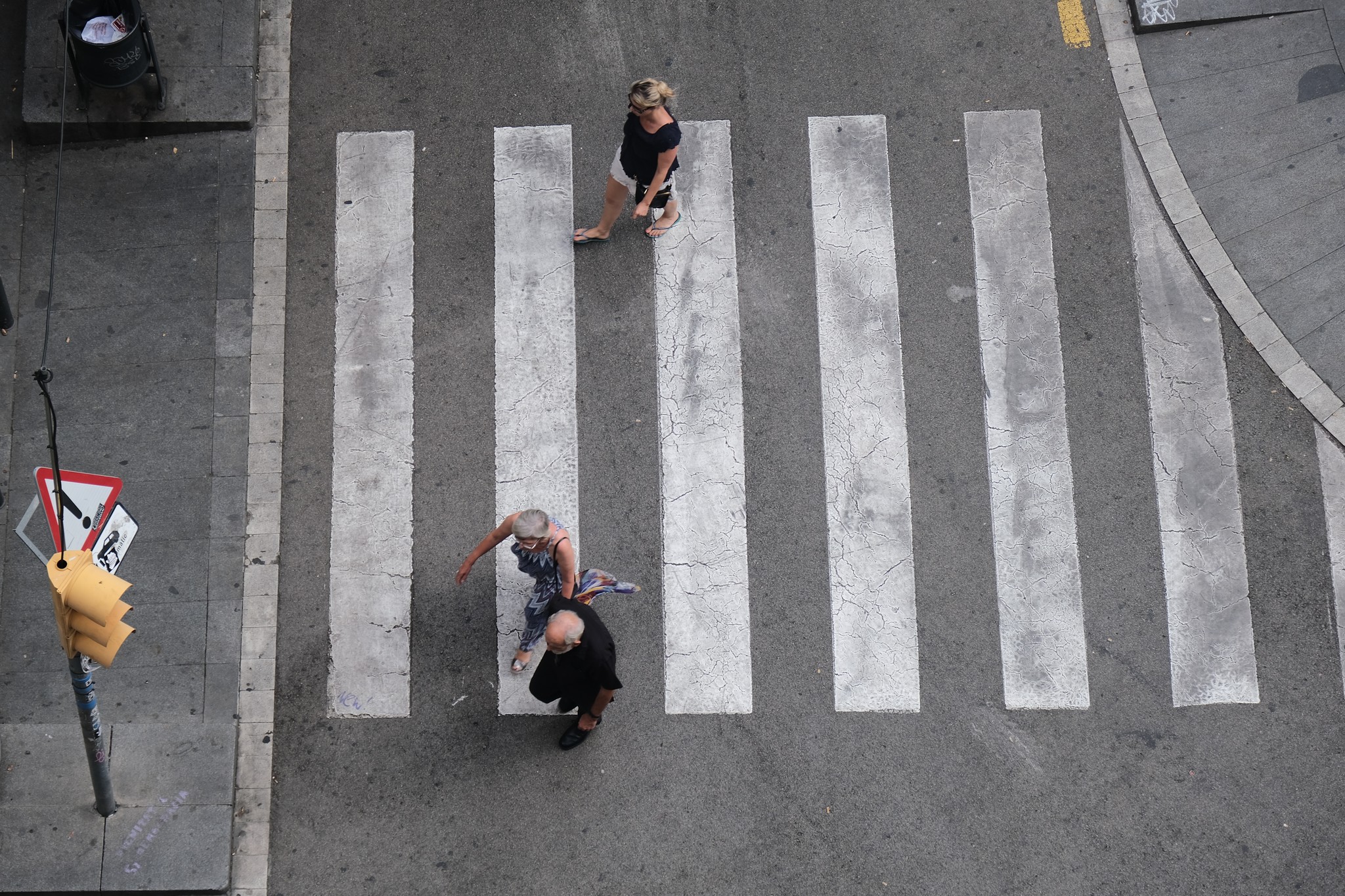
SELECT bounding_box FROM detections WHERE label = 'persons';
[456,509,641,674]
[573,79,681,243]
[528,592,623,751]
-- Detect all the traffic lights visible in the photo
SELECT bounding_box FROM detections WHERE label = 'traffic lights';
[45,549,137,669]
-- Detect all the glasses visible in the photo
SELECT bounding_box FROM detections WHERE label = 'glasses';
[511,533,541,550]
[628,93,656,114]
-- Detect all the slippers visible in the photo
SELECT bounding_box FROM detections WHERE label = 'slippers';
[510,652,532,674]
[646,211,681,238]
[571,228,610,243]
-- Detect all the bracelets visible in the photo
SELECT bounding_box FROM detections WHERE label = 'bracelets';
[587,710,600,718]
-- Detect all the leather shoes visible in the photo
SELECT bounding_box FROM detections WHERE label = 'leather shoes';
[557,696,578,713]
[559,717,591,750]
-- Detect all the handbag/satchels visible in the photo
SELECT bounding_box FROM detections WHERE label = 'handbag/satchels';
[571,582,579,600]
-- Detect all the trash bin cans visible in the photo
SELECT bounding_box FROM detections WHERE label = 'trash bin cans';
[59,0,152,90]
